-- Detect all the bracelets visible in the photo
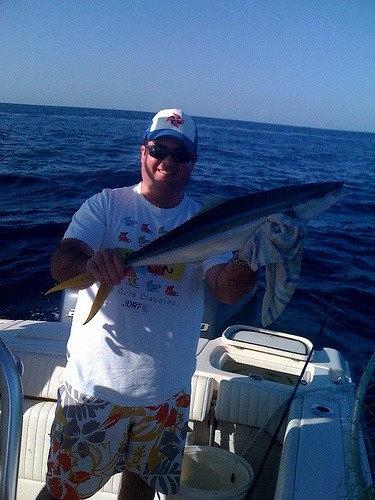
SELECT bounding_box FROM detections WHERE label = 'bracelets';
[235,250,249,266]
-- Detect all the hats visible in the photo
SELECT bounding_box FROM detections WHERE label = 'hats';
[141,109,198,156]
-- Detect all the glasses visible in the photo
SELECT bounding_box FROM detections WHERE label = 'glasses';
[144,145,190,162]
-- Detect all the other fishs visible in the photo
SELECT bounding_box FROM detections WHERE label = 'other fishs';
[43,180,353,325]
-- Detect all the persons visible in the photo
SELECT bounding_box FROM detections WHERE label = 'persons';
[34,108,299,500]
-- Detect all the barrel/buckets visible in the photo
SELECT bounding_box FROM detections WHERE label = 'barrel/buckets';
[157,444,254,500]
